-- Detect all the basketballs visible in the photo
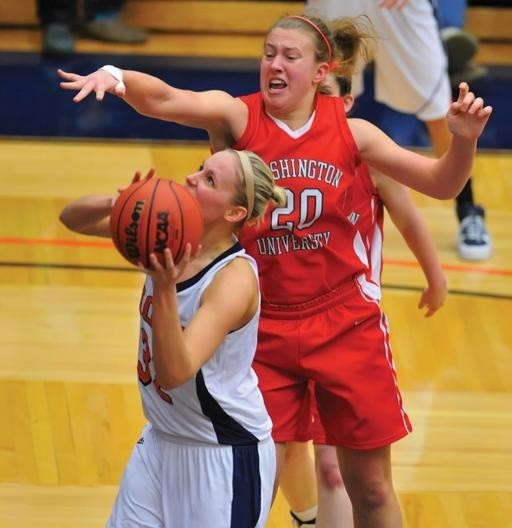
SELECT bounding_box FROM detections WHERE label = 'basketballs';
[110,180,202,271]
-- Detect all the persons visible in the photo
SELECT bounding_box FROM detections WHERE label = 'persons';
[426,0,490,95]
[278,15,450,528]
[59,151,289,528]
[59,11,493,528]
[34,0,146,55]
[305,0,491,262]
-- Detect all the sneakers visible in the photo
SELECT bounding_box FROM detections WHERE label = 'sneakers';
[454,206,492,260]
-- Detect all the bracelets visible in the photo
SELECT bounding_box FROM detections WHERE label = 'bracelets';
[100,61,123,82]
